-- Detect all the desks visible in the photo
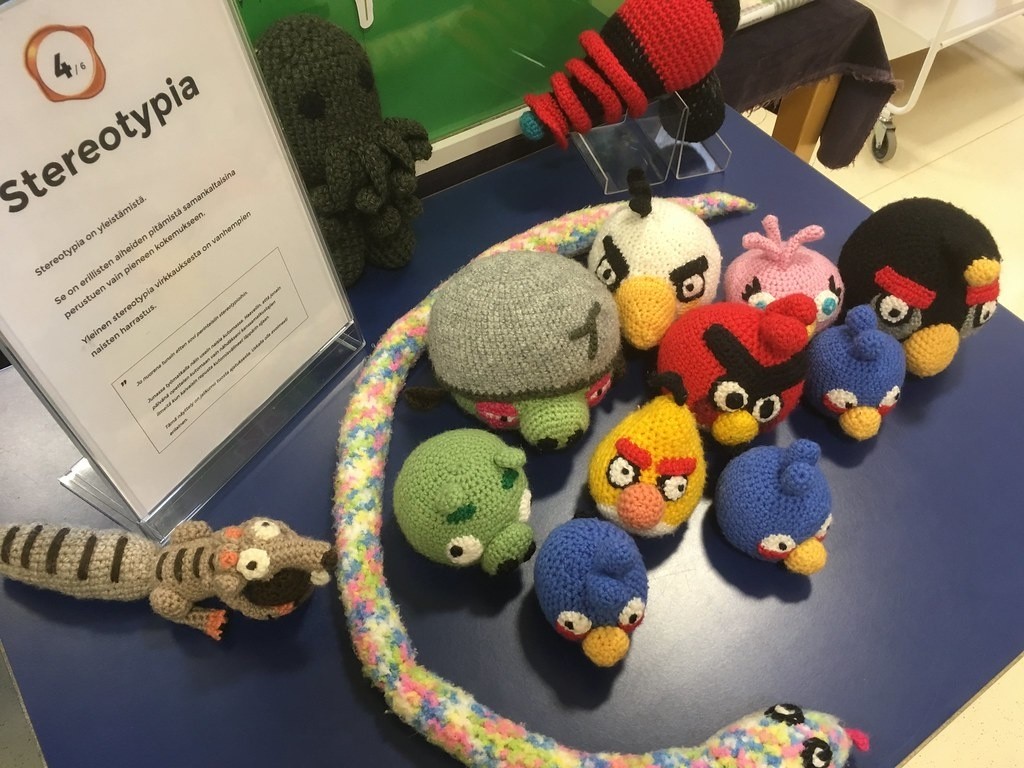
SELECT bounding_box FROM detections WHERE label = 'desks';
[2,111,1024,767]
[230,0,869,194]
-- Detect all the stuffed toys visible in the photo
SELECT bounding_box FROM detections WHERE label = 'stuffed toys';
[809,305,906,442]
[332,191,870,768]
[837,197,1001,378]
[724,215,845,342]
[392,428,537,578]
[517,1,742,149]
[713,439,832,576]
[533,518,648,667]
[657,293,819,446]
[0,517,336,643]
[587,168,721,351]
[252,16,437,289]
[588,370,706,539]
[427,249,622,453]
